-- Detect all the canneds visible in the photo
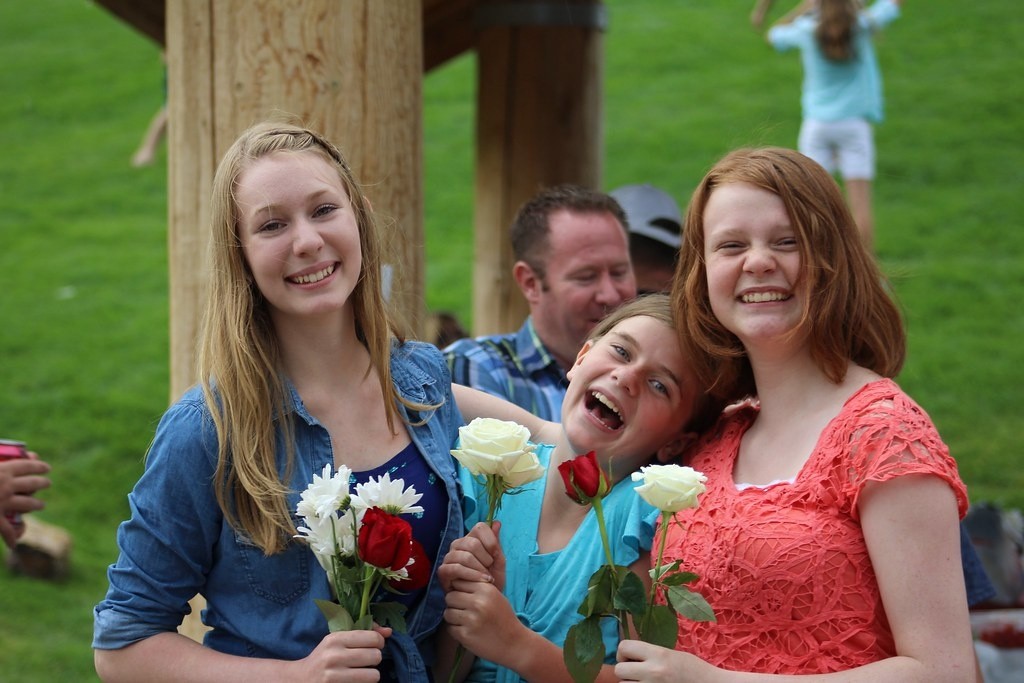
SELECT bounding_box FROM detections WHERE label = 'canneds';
[0,440,30,524]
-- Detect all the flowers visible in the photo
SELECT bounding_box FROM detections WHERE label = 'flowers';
[290,462,432,636]
[450,416,546,529]
[556,452,717,683]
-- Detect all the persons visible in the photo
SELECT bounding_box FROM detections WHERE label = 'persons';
[435,142,979,683]
[766,0,900,246]
[0,460,53,551]
[92,120,466,683]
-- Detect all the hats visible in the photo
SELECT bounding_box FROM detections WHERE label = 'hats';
[611,182,685,247]
